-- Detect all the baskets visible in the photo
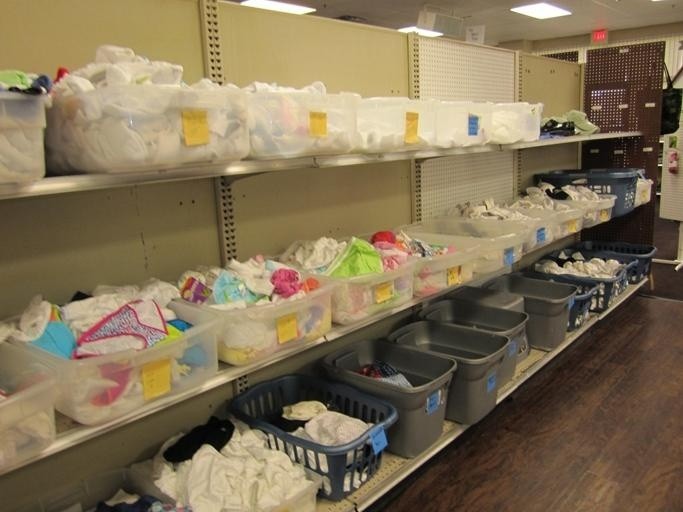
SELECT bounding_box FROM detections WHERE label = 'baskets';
[511,239,657,332]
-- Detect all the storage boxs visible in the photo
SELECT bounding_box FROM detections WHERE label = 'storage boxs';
[277,238,417,325]
[36,470,177,511]
[2,91,45,198]
[247,91,363,161]
[572,240,656,276]
[443,102,490,146]
[417,298,530,362]
[135,457,325,510]
[1,338,60,472]
[481,275,579,352]
[443,286,525,314]
[402,218,529,275]
[530,256,639,313]
[635,180,653,208]
[522,103,543,142]
[160,271,338,368]
[504,208,553,253]
[546,200,584,241]
[44,86,250,175]
[408,98,443,147]
[360,231,478,298]
[569,194,618,230]
[323,338,459,460]
[4,298,224,428]
[385,321,512,426]
[487,102,529,143]
[357,97,410,151]
[520,269,599,331]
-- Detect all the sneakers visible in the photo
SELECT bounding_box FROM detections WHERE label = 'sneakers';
[541,119,574,137]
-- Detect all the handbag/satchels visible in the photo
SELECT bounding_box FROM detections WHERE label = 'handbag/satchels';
[635,88,682,135]
